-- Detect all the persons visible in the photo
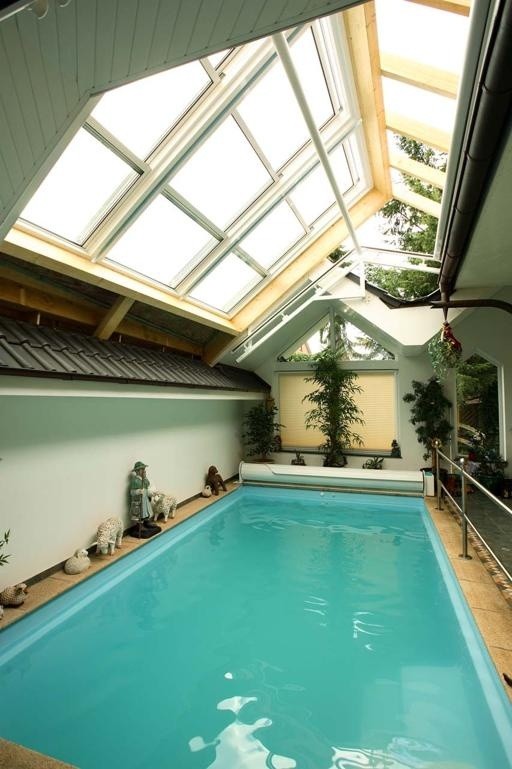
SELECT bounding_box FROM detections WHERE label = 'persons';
[128,461,158,532]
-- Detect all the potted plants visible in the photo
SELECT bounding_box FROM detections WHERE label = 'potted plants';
[469,439,509,500]
[403,376,457,498]
[239,398,284,465]
